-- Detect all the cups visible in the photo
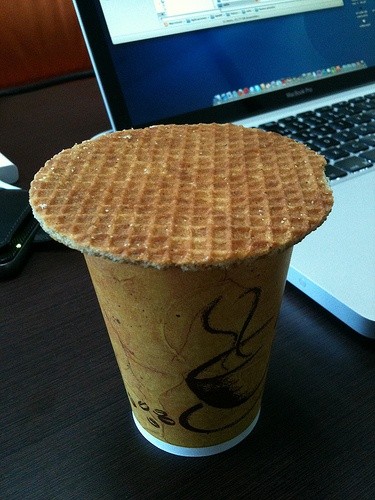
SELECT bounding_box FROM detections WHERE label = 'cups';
[82,246,291,457]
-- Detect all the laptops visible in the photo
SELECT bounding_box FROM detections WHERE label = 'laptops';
[72,0,375,339]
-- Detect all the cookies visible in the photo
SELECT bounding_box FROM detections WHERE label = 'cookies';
[29,123,335,267]
